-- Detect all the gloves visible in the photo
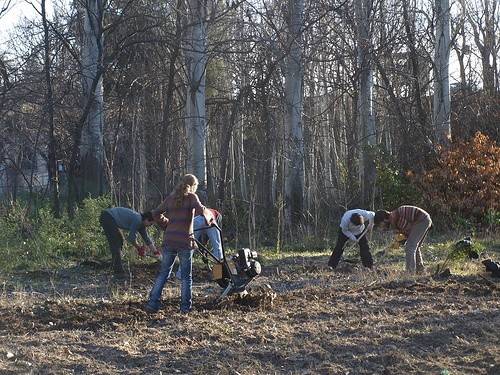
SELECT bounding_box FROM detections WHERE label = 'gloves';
[153,250,162,260]
[136,246,147,257]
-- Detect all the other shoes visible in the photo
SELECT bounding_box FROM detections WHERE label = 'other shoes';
[329,266,336,270]
[363,265,374,273]
[148,299,165,309]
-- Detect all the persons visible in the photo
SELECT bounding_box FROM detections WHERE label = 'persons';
[328,209,376,271]
[175,207,225,279]
[149,173,216,316]
[99,207,162,281]
[374,205,433,276]
